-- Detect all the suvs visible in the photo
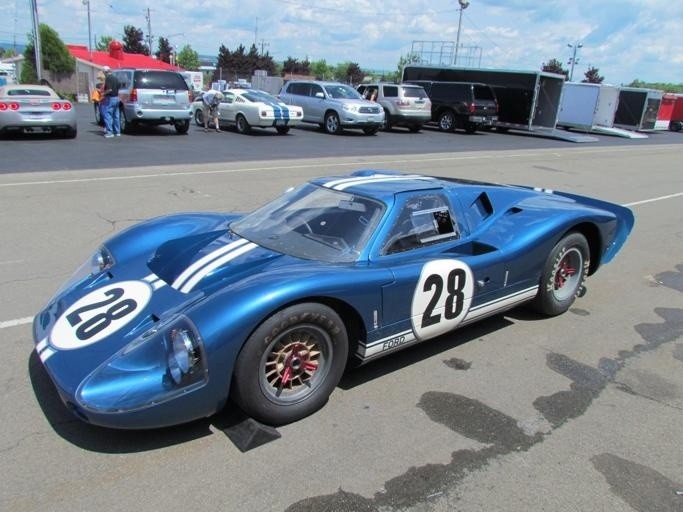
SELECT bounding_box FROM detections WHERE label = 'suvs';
[356,83,432,132]
[280,78,387,135]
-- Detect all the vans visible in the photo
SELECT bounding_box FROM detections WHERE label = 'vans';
[97,67,194,132]
[405,81,501,131]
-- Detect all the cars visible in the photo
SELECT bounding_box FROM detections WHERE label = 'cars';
[192,88,305,134]
[0,84,78,139]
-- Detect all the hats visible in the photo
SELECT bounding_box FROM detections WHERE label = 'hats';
[214,92,223,100]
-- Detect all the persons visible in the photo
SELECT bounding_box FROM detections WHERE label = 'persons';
[96,71,107,134]
[98,65,122,140]
[201,89,224,134]
[365,87,378,103]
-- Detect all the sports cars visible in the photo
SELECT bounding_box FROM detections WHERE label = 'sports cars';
[34,167,634,430]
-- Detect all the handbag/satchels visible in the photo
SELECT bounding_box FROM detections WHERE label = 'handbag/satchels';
[91,89,103,102]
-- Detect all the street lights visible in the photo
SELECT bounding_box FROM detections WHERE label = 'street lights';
[566,44,583,80]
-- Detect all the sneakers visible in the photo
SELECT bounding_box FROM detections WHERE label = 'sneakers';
[103,126,120,138]
[204,129,222,134]
